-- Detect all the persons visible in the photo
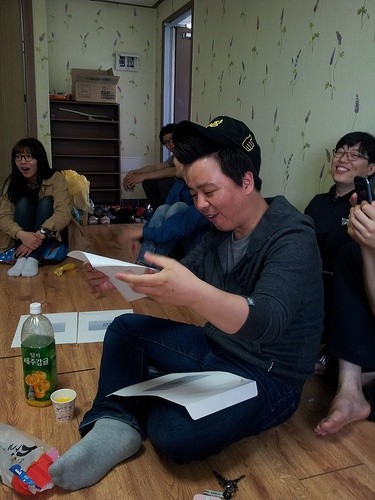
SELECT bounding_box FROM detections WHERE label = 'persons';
[304,132,375,436]
[0,138,73,277]
[125,122,209,267]
[46,116,328,493]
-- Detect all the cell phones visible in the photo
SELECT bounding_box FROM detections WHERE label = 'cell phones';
[354,175,372,219]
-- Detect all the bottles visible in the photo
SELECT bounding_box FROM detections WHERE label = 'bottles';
[20,302,57,407]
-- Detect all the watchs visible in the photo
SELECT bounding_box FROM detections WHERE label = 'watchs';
[40,229,48,238]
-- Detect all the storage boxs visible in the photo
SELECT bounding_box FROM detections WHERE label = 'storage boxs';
[72,67,119,104]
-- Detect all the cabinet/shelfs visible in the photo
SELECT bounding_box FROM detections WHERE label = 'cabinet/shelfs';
[50,100,121,205]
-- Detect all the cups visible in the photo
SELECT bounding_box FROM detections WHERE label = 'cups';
[50,388,76,422]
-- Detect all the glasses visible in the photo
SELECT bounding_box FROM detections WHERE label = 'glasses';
[15,154,34,162]
[333,147,370,162]
[163,137,173,147]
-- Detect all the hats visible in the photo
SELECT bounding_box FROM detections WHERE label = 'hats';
[159,115,261,176]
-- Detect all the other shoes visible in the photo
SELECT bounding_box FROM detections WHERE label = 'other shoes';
[88,212,111,225]
